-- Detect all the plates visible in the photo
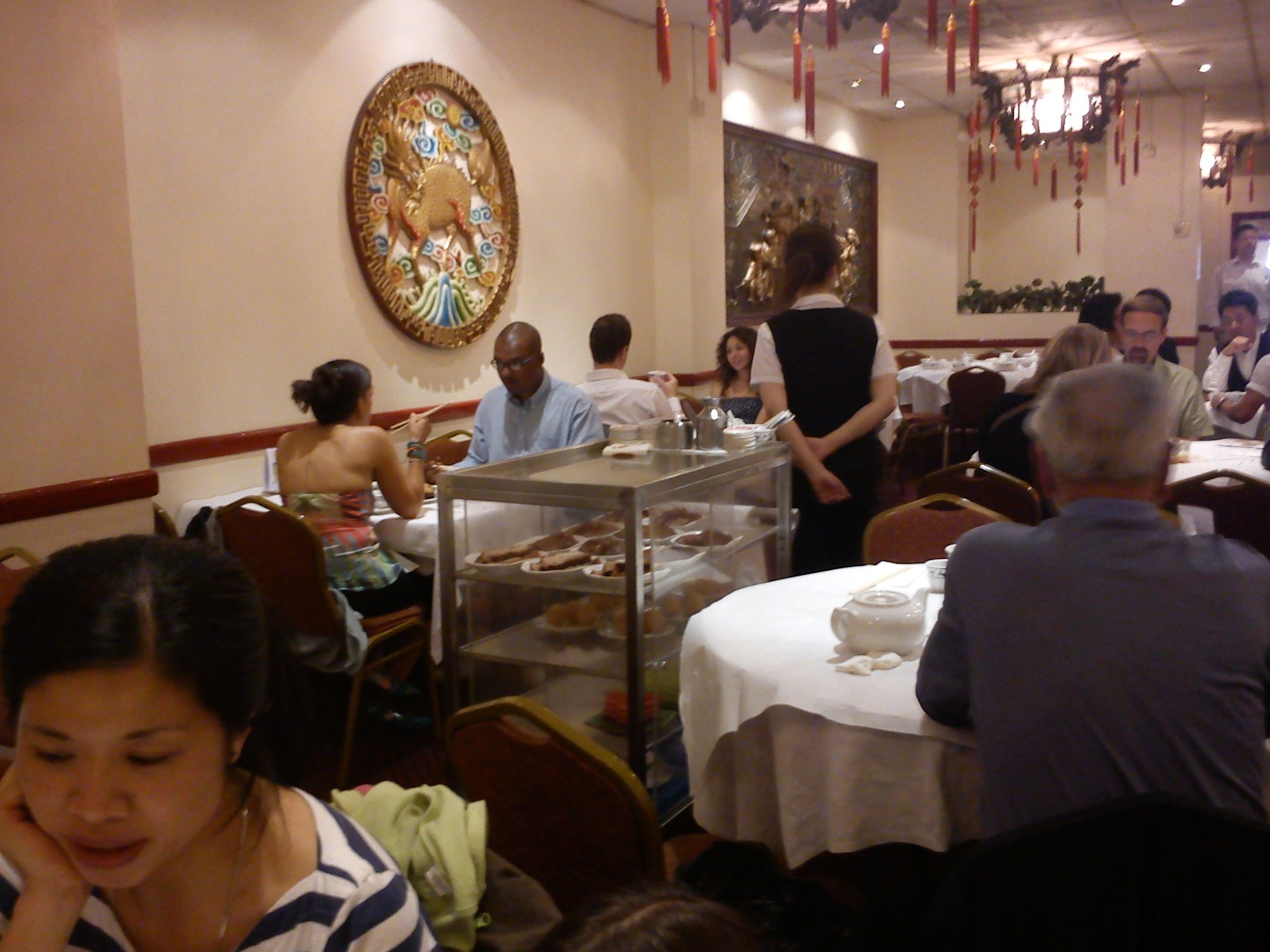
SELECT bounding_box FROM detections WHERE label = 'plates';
[639,510,705,527]
[670,530,744,552]
[463,549,546,567]
[578,665,689,810]
[537,570,731,640]
[561,523,624,540]
[368,465,461,514]
[595,508,650,527]
[580,558,670,583]
[569,539,651,560]
[637,545,706,564]
[521,552,606,579]
[610,526,685,542]
[515,534,588,557]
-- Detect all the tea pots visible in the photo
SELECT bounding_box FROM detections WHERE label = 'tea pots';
[830,585,931,658]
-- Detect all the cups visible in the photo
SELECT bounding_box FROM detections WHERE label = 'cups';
[945,544,957,556]
[925,558,948,591]
[1177,440,1192,462]
[647,370,667,386]
[657,418,694,449]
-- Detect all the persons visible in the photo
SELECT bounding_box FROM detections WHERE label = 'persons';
[749,220,898,577]
[1119,295,1216,441]
[914,359,1268,835]
[1200,288,1270,393]
[1077,291,1123,346]
[275,358,434,723]
[974,323,1111,519]
[1212,223,1270,338]
[708,325,769,424]
[573,312,689,434]
[1134,287,1179,365]
[2,532,442,952]
[422,320,607,484]
[1211,353,1270,425]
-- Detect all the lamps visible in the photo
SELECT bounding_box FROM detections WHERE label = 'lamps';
[963,45,1148,256]
[1201,124,1263,206]
[649,0,990,139]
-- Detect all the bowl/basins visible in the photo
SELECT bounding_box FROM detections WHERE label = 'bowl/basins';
[920,352,1040,371]
[606,416,661,447]
[722,424,776,454]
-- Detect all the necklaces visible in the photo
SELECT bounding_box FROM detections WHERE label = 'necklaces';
[216,796,250,950]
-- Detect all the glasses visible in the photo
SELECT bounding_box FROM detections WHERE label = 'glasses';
[490,354,537,372]
[1122,328,1163,341]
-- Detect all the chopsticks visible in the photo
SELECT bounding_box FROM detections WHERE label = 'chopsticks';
[390,400,452,435]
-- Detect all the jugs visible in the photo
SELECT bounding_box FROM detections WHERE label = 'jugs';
[695,396,737,449]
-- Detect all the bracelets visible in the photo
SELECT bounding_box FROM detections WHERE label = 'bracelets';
[407,441,428,460]
[1216,397,1227,410]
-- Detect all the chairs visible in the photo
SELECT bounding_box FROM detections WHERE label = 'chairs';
[190,346,1266,952]
